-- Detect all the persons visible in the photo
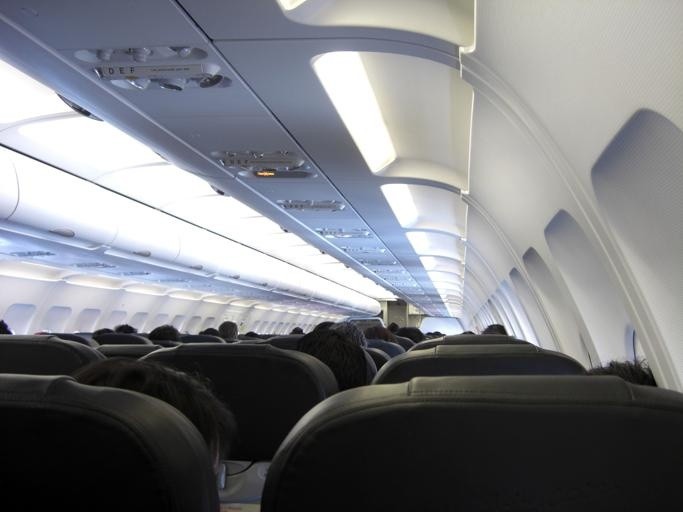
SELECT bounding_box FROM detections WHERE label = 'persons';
[583,357,657,389]
[94,320,442,392]
[480,323,507,336]
[76,355,239,491]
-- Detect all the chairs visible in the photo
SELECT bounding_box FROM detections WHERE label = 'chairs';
[0,318,683,512]
